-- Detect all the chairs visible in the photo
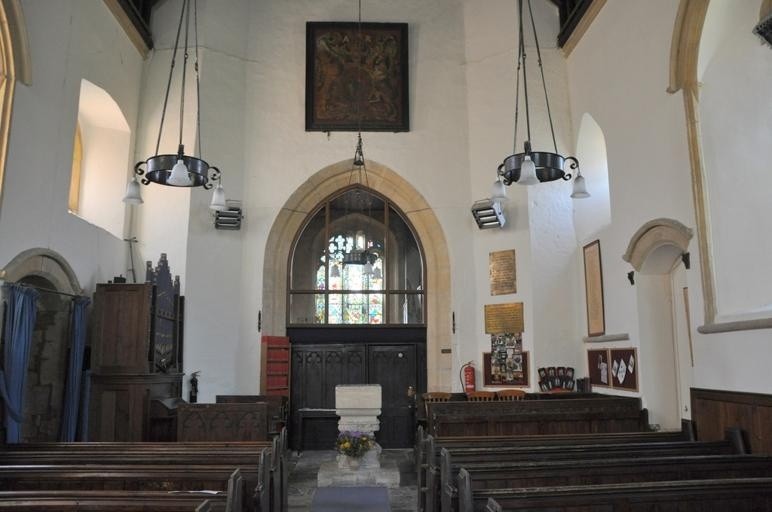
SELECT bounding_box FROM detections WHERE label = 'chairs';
[422,390,526,432]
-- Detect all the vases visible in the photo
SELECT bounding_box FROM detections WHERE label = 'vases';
[349,458,360,468]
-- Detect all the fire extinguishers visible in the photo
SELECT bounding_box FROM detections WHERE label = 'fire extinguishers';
[189,371,202,403]
[458,360,476,396]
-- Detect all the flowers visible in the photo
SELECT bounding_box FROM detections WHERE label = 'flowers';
[334,432,371,457]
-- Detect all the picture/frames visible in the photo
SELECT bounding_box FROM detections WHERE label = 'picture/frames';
[306,22,409,131]
[583,239,605,337]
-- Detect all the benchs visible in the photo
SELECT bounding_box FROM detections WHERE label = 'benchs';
[0,426,290,511]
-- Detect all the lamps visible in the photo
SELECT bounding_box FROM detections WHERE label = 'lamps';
[123,1,229,213]
[492,1,591,204]
[470,198,506,230]
[331,0,382,279]
[215,200,244,230]
